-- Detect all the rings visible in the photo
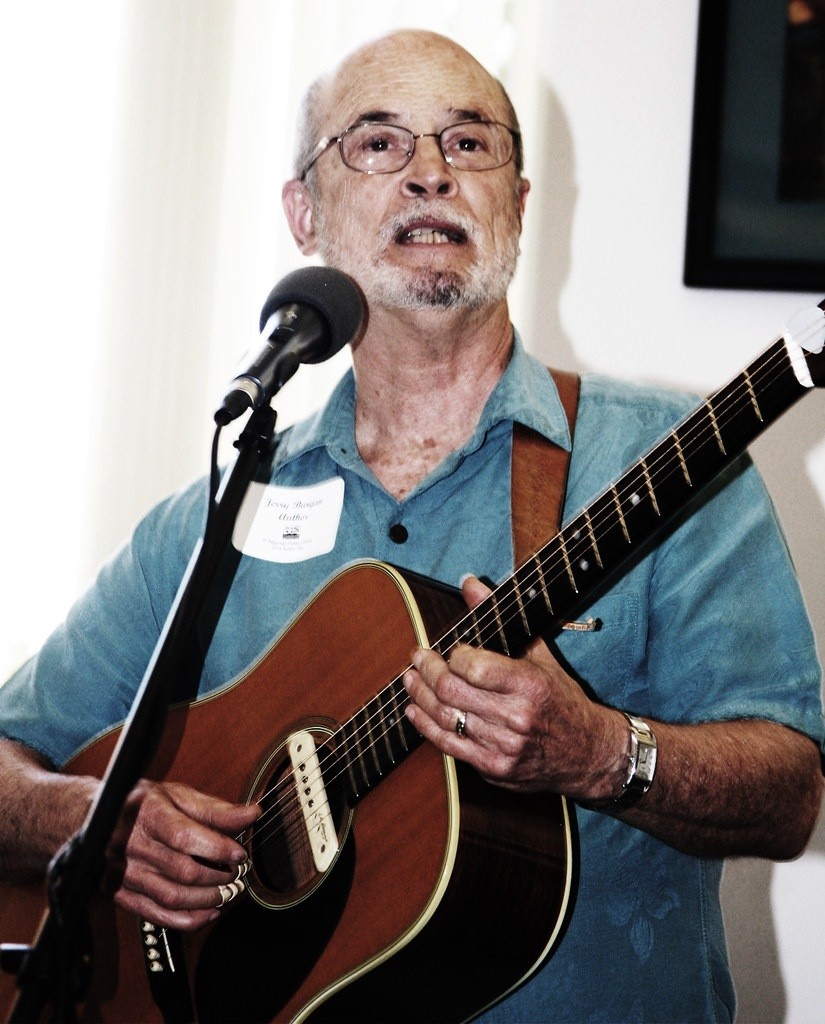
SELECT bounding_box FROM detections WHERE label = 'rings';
[455,709,470,740]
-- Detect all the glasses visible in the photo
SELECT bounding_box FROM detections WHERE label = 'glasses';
[298,123,525,183]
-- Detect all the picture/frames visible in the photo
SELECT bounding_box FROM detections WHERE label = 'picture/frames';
[684,2,825,291]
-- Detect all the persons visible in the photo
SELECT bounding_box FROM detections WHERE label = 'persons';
[1,28,825,1024]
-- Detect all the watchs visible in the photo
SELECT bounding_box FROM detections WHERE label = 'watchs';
[573,709,657,814]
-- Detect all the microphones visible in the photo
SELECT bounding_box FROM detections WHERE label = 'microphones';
[213,266,364,424]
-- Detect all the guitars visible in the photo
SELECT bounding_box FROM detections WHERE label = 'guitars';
[0,296,825,1024]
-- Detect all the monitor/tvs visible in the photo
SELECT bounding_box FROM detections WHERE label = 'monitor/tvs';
[681,1,825,291]
